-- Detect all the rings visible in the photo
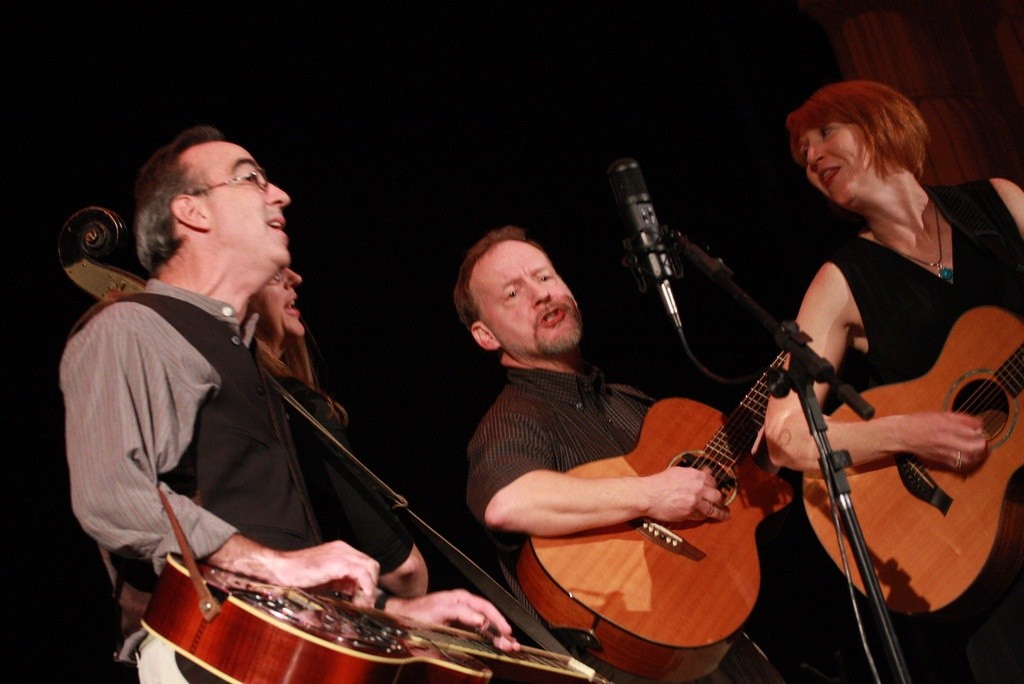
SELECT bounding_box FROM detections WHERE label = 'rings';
[957,453,962,468]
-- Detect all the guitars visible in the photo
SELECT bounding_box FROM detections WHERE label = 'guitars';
[800,304,1024,620]
[136,549,617,684]
[508,347,790,682]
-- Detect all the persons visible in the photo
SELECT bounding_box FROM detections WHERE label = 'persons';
[453,227,784,684]
[766,80,1024,684]
[59,125,519,684]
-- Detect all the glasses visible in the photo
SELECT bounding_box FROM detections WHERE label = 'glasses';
[271,270,282,282]
[190,168,270,191]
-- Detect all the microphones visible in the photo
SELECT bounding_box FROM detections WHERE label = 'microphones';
[609,157,681,331]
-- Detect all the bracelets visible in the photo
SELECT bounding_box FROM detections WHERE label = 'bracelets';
[374,594,392,610]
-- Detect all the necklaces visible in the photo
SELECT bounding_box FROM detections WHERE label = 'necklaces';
[872,204,953,281]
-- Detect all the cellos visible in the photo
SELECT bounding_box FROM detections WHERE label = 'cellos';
[53,204,147,301]
